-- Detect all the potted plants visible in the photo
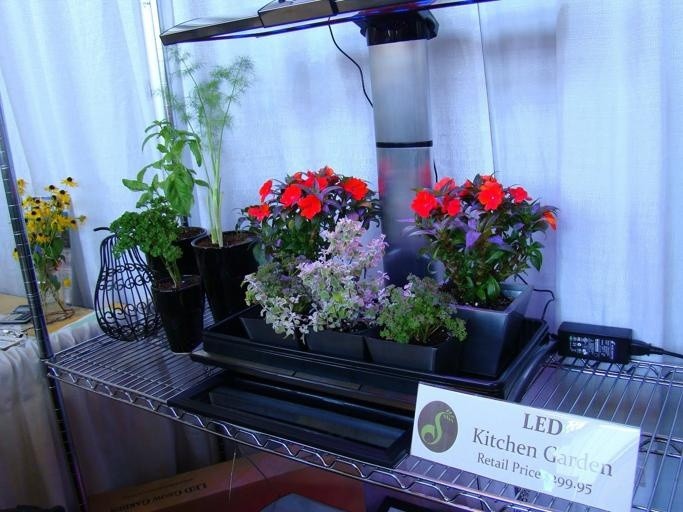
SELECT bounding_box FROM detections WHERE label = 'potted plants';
[109,197,205,352]
[146,48,256,323]
[122,116,211,275]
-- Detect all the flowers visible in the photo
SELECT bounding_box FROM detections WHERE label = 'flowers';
[13,177,87,324]
[241,164,390,335]
[390,173,560,304]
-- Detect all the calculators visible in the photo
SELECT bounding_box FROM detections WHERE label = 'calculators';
[0,305,33,323]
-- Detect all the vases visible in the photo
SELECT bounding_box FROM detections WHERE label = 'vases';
[301,328,365,359]
[452,283,534,375]
[238,310,300,349]
[41,274,75,323]
[365,337,466,374]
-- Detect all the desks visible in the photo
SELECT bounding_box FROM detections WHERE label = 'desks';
[1,294,97,510]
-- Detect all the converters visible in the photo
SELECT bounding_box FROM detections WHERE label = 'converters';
[557,321,633,366]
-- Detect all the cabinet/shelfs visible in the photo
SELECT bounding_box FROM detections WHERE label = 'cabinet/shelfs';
[0,98,683,512]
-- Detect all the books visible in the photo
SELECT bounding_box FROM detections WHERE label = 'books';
[87,444,368,512]
